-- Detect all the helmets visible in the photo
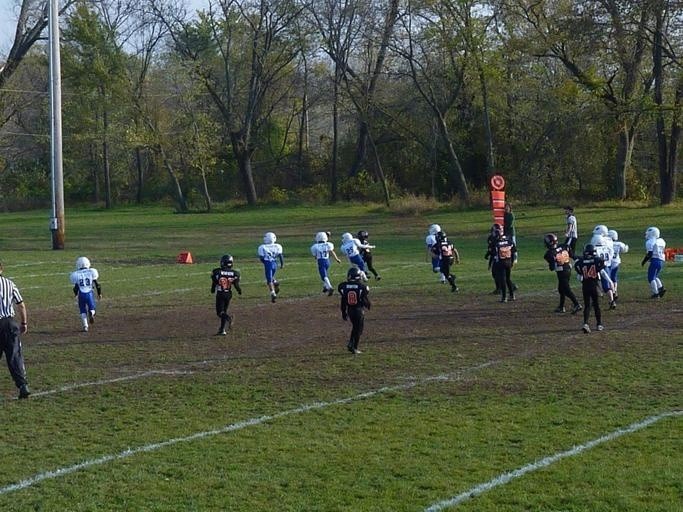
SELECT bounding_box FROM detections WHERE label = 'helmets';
[645,227,660,240]
[347,267,360,281]
[264,232,276,244]
[220,255,234,268]
[544,234,559,247]
[315,232,328,242]
[436,231,447,241]
[608,230,618,242]
[583,243,596,257]
[358,231,368,239]
[591,235,606,247]
[342,232,353,243]
[491,223,504,236]
[76,257,90,269]
[428,224,441,235]
[592,225,609,237]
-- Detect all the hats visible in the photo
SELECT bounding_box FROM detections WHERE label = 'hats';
[564,205,574,211]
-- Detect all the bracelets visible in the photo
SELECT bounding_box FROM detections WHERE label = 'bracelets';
[21,322,28,325]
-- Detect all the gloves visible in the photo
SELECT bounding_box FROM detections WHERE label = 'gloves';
[365,301,370,310]
[211,288,215,293]
[237,290,242,295]
[342,312,348,321]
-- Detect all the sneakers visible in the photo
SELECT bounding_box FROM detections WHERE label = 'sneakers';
[375,275,381,280]
[273,280,279,294]
[328,287,336,296]
[658,286,667,298]
[322,286,328,292]
[228,313,235,329]
[513,285,518,291]
[651,294,659,299]
[608,300,617,309]
[554,306,566,313]
[509,295,515,300]
[216,329,227,336]
[451,286,456,292]
[614,292,619,300]
[572,303,583,313]
[450,272,456,280]
[497,298,508,302]
[83,326,88,331]
[492,288,501,294]
[89,310,96,323]
[348,343,353,352]
[352,348,361,353]
[598,325,604,331]
[271,293,277,303]
[582,324,591,333]
[18,384,31,399]
[441,280,445,283]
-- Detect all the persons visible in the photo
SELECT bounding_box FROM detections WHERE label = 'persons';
[570,242,615,333]
[591,235,617,310]
[211,254,241,336]
[358,230,382,281]
[337,266,372,353]
[641,226,667,299]
[492,222,516,303]
[564,204,578,253]
[543,233,582,314]
[593,225,614,295]
[340,232,376,277]
[425,223,447,284]
[428,232,459,293]
[608,229,629,301]
[488,234,517,294]
[0,261,31,399]
[504,203,519,263]
[257,231,285,304]
[69,255,103,332]
[311,230,341,296]
